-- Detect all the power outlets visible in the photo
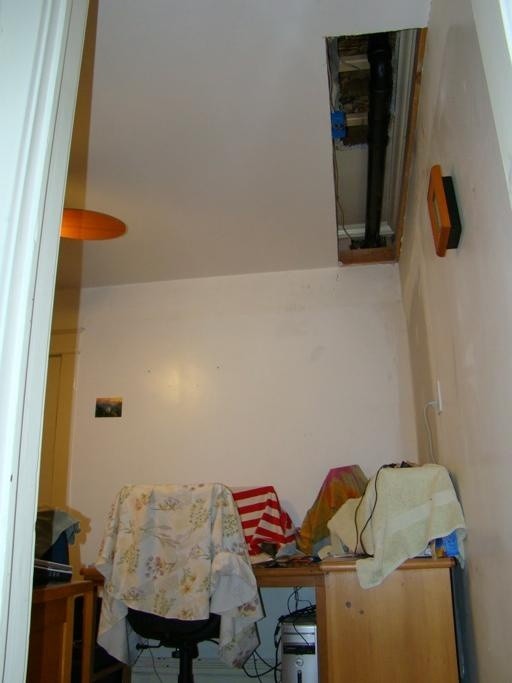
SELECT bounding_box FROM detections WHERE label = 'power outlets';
[434,379,442,414]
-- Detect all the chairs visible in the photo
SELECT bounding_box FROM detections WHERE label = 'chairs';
[125,482,229,683]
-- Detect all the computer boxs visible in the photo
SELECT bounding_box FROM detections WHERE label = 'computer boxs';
[281,605,319,683]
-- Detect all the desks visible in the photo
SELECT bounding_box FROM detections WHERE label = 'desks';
[426,165,450,258]
